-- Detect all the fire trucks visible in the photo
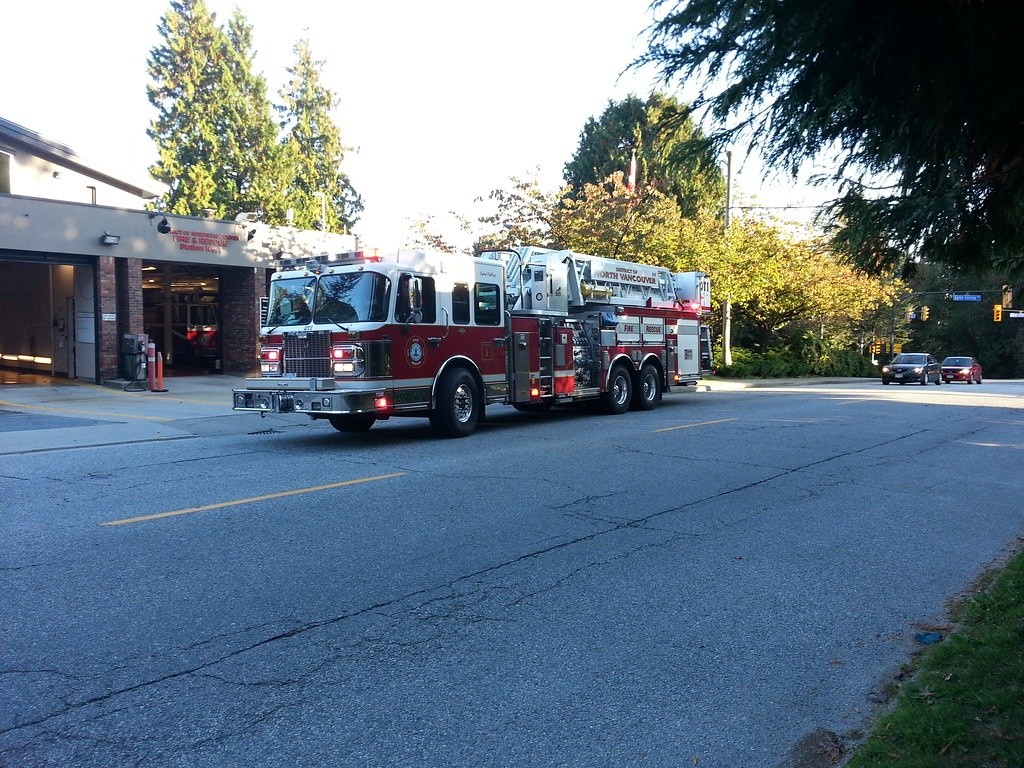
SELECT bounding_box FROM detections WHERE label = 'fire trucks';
[230,243,716,439]
[143,287,223,372]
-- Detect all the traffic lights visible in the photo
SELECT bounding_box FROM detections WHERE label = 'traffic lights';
[922,305,929,322]
[994,305,1001,321]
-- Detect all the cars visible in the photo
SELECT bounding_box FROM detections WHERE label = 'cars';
[939,356,983,384]
[882,352,942,385]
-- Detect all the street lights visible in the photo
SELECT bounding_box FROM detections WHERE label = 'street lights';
[312,192,326,231]
